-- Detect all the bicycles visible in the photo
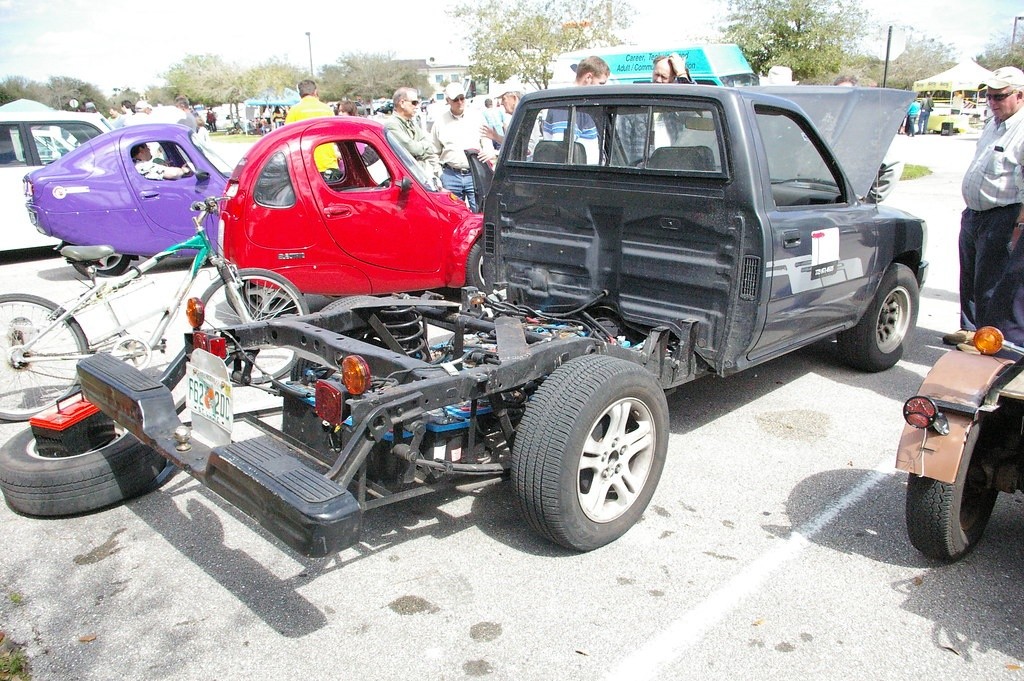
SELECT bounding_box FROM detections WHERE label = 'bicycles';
[0,194,313,422]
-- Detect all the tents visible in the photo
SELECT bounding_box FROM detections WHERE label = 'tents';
[244,87,301,136]
[913,55,996,92]
[0,99,57,113]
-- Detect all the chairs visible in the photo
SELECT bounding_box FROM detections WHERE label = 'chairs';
[648,145,716,171]
[586,149,603,164]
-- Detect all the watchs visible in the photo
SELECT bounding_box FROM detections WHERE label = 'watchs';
[1014,222,1024,230]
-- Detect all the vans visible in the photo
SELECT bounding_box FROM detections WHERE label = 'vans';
[0,109,118,255]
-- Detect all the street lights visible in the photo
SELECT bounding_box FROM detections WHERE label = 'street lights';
[305,31,314,78]
[1011,16,1024,44]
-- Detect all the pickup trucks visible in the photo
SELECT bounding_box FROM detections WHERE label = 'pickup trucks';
[73,82,931,556]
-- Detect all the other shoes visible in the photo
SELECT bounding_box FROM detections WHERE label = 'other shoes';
[943,331,980,346]
[957,341,980,355]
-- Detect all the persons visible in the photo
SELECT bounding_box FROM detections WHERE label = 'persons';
[898,99,920,137]
[256,105,290,135]
[543,55,606,167]
[357,94,362,102]
[337,100,366,172]
[107,94,217,144]
[610,52,700,166]
[284,80,344,183]
[943,67,1024,355]
[951,91,965,133]
[915,92,934,135]
[833,74,904,203]
[385,82,540,210]
[758,66,799,86]
[130,142,190,181]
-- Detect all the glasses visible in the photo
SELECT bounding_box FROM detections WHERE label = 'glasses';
[404,99,418,106]
[449,96,465,103]
[986,90,1021,102]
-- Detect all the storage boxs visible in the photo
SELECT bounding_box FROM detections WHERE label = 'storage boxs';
[30,388,116,458]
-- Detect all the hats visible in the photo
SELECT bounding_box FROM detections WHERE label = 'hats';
[759,66,799,87]
[495,82,521,99]
[978,66,1024,91]
[446,82,466,100]
